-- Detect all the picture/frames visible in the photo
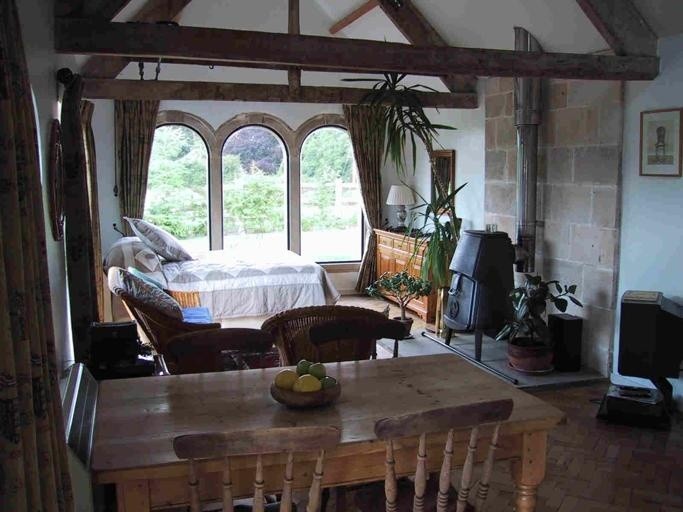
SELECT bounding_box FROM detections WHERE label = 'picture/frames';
[639,107,683,177]
[429,150,456,216]
[50,118,64,242]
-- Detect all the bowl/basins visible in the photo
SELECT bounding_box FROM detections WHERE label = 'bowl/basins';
[269,376,342,408]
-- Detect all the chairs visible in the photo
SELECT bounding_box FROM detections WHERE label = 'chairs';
[107,266,221,376]
[166,328,274,375]
[172,425,341,512]
[260,306,387,371]
[373,397,515,512]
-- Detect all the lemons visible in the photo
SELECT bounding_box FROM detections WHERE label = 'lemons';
[296,359,312,376]
[309,363,326,380]
[320,376,336,390]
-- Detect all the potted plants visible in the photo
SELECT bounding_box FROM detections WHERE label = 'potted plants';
[497,273,584,377]
[365,271,432,340]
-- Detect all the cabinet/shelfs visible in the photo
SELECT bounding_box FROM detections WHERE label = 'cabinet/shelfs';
[373,227,457,324]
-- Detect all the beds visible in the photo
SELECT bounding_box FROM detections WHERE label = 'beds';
[102,236,340,320]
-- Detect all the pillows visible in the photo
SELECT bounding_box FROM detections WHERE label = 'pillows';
[123,216,195,262]
[107,267,183,330]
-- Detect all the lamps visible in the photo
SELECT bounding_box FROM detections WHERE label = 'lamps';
[384,185,416,233]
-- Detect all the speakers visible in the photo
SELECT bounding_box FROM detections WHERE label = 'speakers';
[547,313,583,371]
